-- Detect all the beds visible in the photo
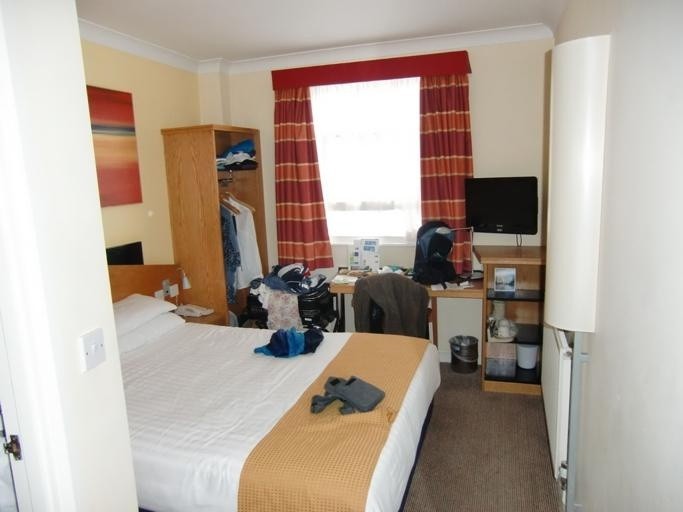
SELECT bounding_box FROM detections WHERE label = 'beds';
[95,263,441,512]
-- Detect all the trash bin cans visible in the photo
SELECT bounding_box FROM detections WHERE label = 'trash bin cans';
[449,335,479,374]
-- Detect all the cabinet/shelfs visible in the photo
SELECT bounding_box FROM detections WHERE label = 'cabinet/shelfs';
[161,124,272,325]
[476,243,543,396]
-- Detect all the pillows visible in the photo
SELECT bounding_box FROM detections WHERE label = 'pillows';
[110,292,175,335]
[117,310,187,352]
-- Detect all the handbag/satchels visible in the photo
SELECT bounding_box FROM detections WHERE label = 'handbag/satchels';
[310,375,385,415]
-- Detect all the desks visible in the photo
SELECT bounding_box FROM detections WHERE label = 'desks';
[334,259,485,331]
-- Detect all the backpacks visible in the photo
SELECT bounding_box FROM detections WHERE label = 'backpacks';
[412,220,457,289]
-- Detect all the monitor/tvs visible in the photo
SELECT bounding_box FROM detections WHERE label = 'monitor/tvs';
[464,176,538,235]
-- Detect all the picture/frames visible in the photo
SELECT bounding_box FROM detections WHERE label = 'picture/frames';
[494,267,516,292]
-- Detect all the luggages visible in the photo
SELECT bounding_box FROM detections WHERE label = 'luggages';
[247,284,335,328]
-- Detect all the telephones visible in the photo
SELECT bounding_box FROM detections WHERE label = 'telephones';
[173,303,210,319]
